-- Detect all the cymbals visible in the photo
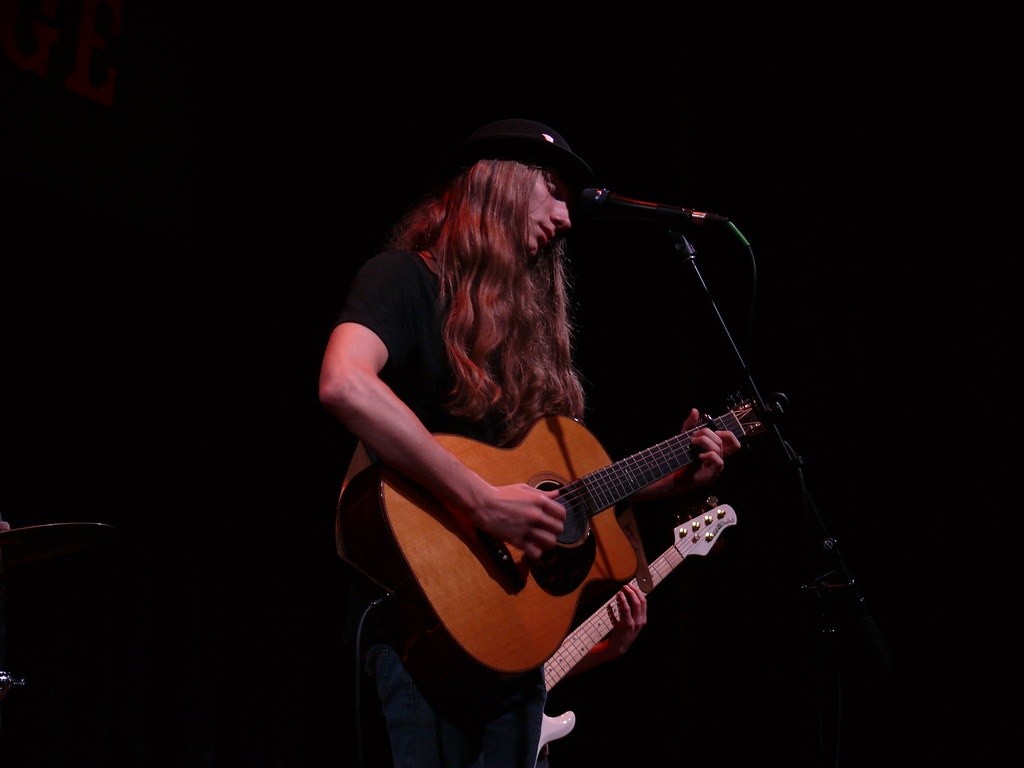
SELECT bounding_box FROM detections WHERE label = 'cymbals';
[0,520,114,553]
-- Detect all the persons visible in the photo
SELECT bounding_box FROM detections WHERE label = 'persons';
[553,583,651,673]
[320,121,736,768]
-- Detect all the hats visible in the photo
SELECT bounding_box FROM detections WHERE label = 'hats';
[465,118,596,195]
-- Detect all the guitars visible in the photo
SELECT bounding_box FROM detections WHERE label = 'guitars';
[337,392,769,686]
[527,490,738,767]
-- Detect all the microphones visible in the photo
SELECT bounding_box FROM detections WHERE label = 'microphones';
[583,188,729,227]
